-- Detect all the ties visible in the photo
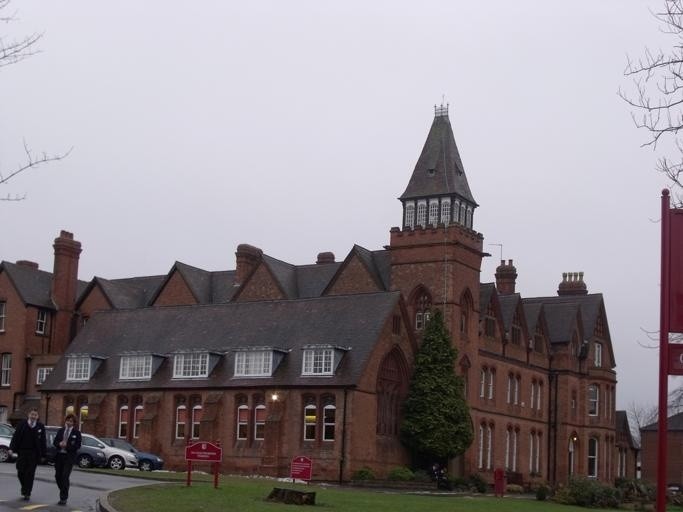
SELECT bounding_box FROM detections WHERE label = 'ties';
[63,428,69,441]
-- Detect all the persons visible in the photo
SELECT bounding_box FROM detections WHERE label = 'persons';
[50,413,82,506]
[7,408,47,502]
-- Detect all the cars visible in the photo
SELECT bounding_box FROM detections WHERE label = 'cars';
[0,422,165,472]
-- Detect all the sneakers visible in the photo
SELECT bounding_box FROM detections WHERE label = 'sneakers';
[24,495,67,506]
